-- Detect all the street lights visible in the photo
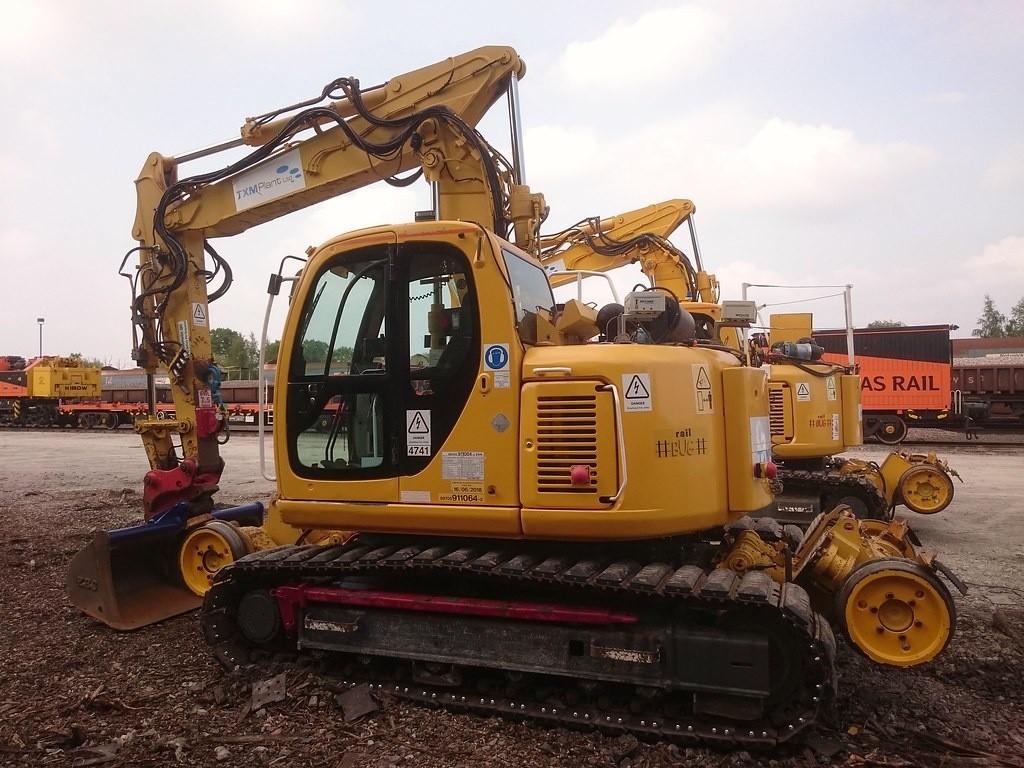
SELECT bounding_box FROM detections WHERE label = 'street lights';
[37,317,45,359]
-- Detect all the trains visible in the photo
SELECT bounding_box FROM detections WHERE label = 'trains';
[0,355,350,434]
[751,322,1024,445]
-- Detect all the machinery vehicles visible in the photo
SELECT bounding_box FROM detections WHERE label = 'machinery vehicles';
[65,44,957,750]
